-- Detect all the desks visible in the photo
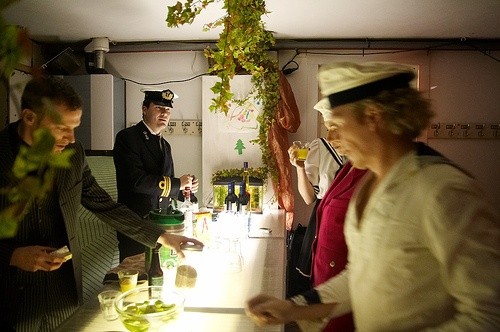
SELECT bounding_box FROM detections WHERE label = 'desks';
[54,208,286,332]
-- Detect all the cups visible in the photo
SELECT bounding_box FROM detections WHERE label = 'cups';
[117,269,139,294]
[97,290,122,320]
[293,140,309,161]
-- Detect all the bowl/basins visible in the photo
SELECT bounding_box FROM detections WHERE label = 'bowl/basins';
[114,286,185,332]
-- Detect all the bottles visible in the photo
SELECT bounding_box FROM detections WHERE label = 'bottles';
[181,187,198,238]
[224,162,250,213]
[148,248,164,298]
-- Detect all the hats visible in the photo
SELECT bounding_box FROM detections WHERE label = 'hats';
[317,60,419,109]
[139,84,181,111]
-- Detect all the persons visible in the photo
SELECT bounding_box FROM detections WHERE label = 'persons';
[310,160,368,288]
[0,75,204,332]
[246,61,500,332]
[287,138,346,295]
[113,87,199,264]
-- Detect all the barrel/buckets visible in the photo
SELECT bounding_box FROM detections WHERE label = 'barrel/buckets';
[146,208,185,262]
